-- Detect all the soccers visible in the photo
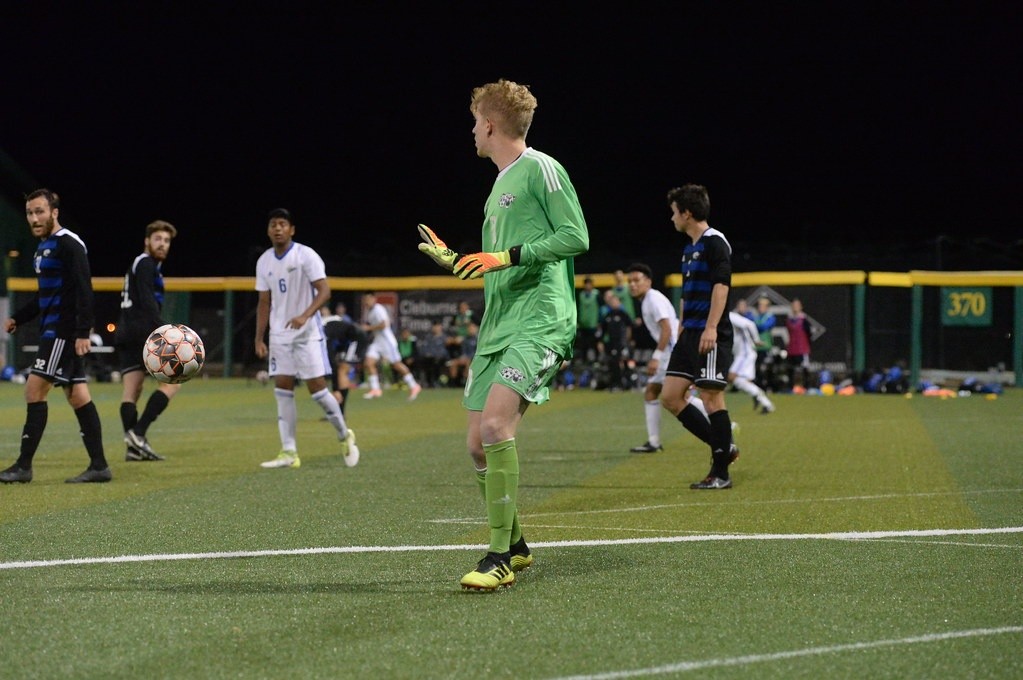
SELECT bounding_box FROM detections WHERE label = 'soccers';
[142,322,206,384]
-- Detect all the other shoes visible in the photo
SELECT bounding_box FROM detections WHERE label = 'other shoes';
[760,405,776,415]
[752,395,760,411]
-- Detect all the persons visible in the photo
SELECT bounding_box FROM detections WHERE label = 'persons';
[317,269,776,419]
[417,80,588,589]
[728,311,776,414]
[660,183,740,490]
[255,207,360,468]
[785,297,815,390]
[626,264,738,453]
[0,189,113,483]
[353,290,422,400]
[114,221,182,460]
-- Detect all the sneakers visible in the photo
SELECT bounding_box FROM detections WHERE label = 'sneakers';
[408,384,421,400]
[510,536,532,571]
[711,444,739,465]
[64,467,112,483]
[690,474,733,490]
[126,448,143,460]
[339,429,359,467]
[460,552,515,592]
[730,422,740,441]
[0,462,32,483]
[363,390,382,399]
[123,429,165,461]
[260,449,301,469]
[630,442,663,453]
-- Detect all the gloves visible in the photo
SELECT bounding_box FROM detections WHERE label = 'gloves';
[453,249,513,280]
[417,224,458,271]
